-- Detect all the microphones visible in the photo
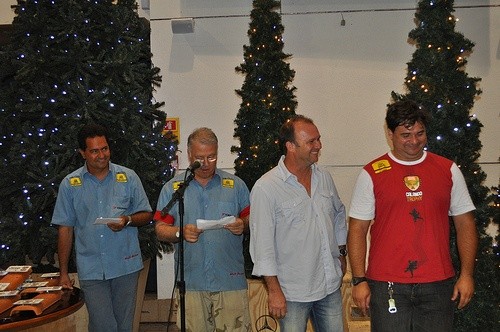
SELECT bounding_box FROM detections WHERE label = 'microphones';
[187,159,203,171]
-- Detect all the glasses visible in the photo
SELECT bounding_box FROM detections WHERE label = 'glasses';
[195,158,216,163]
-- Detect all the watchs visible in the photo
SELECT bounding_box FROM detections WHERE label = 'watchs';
[125,215,132,227]
[338,249,347,257]
[352,277,368,286]
[176,227,180,237]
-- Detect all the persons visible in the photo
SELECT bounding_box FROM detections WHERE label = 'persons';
[347,102,476,332]
[153,128,251,332]
[249,117,348,332]
[51,125,152,332]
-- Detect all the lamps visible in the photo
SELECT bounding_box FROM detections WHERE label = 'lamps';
[171,18,195,34]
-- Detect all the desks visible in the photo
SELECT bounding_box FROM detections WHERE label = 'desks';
[0,286,85,332]
